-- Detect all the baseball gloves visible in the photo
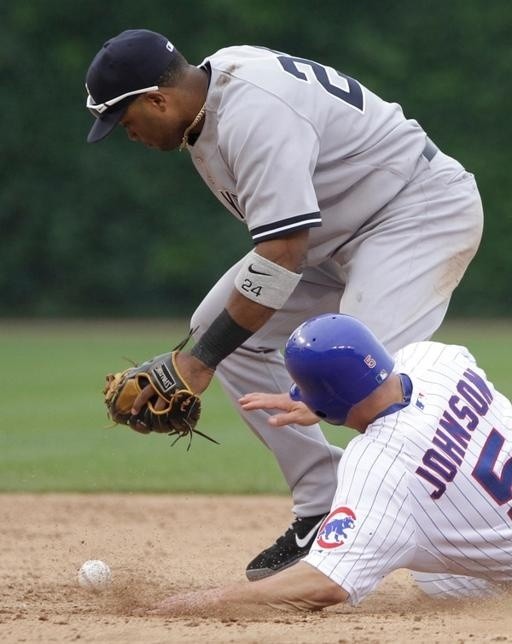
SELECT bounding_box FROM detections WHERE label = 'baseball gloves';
[103,350,200,436]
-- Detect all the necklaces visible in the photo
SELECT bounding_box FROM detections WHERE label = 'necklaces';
[178,100,207,152]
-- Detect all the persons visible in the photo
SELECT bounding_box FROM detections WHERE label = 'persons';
[81,25,487,583]
[130,306,510,627]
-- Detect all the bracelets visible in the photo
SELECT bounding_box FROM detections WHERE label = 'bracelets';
[190,307,254,375]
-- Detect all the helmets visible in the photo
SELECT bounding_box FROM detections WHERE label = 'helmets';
[283,312,396,426]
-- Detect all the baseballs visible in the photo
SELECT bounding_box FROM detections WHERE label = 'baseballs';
[77,559,112,594]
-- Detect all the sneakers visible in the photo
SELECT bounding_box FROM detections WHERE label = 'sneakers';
[245,512,331,581]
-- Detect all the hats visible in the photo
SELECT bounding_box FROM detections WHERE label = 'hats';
[84,29,179,144]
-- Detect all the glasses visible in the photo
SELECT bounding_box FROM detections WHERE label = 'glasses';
[86,85,159,121]
